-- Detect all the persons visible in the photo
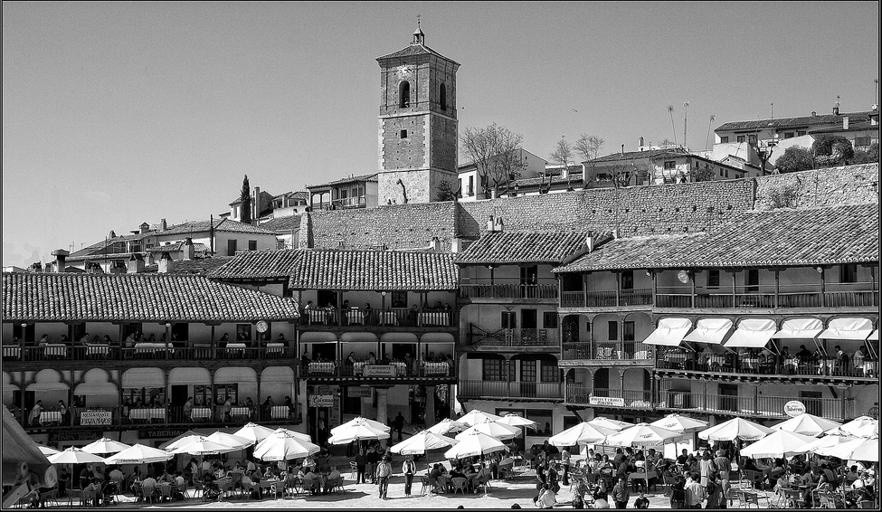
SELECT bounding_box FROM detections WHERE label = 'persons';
[1,297,882,511]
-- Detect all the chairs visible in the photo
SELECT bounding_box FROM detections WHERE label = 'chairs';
[567,460,666,490]
[596,346,613,359]
[420,450,536,495]
[728,458,879,508]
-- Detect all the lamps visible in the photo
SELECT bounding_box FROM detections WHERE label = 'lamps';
[256,320,269,333]
[677,269,691,284]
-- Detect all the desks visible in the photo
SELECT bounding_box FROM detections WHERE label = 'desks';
[37,404,290,419]
[664,353,879,376]
[308,359,448,375]
[41,341,285,355]
[308,307,449,326]
